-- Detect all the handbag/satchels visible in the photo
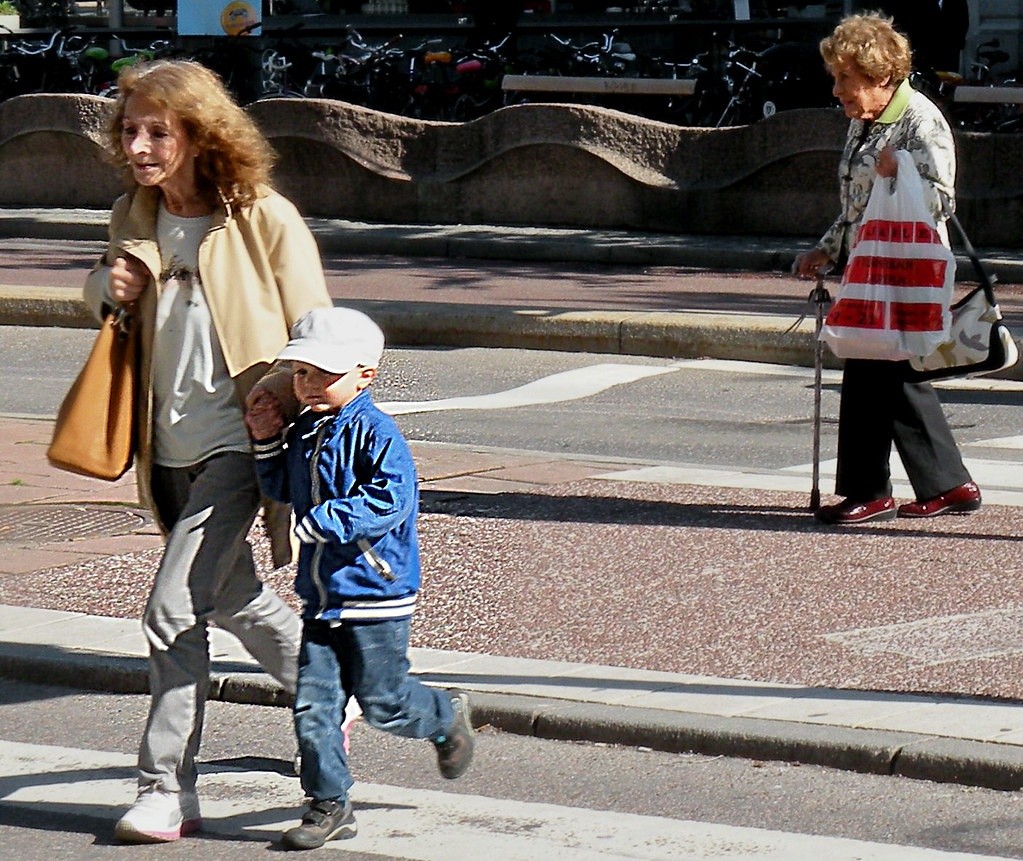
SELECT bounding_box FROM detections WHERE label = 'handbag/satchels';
[45,301,143,481]
[902,278,1019,385]
[819,148,955,360]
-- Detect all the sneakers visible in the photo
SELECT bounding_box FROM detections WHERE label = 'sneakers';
[281,801,359,850]
[294,696,363,775]
[429,688,474,778]
[114,787,200,843]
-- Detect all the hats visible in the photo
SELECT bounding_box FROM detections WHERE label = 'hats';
[277,307,385,376]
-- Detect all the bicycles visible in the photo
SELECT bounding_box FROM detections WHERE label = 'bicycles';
[0,0,1023,132]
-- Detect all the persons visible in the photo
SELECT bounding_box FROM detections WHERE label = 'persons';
[789,14,984,521]
[83,60,340,842]
[243,308,477,849]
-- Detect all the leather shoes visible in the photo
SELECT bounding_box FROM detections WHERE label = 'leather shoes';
[899,480,983,517]
[813,498,897,524]
